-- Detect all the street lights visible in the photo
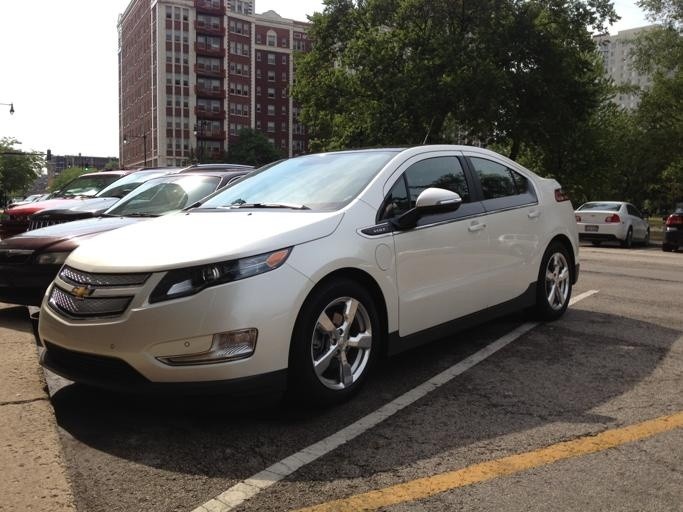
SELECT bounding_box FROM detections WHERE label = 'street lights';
[192,120,204,164]
[0,103,16,115]
[122,133,147,166]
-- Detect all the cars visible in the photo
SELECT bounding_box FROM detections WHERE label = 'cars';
[24,143,584,415]
[661,201,683,252]
[572,199,652,246]
[0,157,254,312]
